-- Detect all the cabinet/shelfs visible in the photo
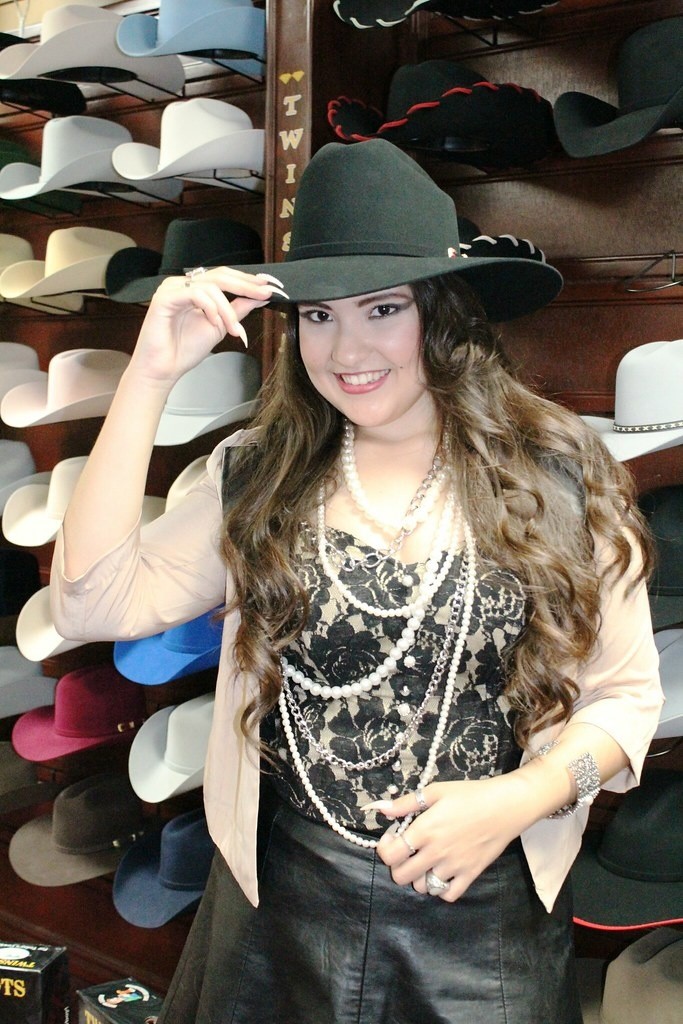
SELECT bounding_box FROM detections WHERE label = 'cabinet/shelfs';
[0,0,683,1024]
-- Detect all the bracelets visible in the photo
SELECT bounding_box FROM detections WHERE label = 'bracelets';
[523,740,601,818]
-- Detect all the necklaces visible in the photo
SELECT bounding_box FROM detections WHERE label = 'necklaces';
[278,418,477,846]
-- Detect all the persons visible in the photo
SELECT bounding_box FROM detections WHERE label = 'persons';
[49,139,665,1024]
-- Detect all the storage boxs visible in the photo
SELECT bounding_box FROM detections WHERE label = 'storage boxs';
[0,941,71,1024]
[76,978,165,1024]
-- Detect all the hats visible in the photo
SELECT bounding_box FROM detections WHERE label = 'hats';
[0,113,187,203]
[326,58,555,165]
[332,0,559,33]
[0,31,88,115]
[561,927,683,1024]
[573,337,683,466]
[103,218,262,304]
[0,342,49,407]
[551,14,683,159]
[556,769,682,930]
[653,628,683,742]
[181,136,567,321]
[0,234,84,316]
[0,347,133,429]
[0,5,187,100]
[0,140,84,214]
[151,349,267,446]
[115,0,269,79]
[633,483,683,631]
[111,96,266,194]
[0,440,228,928]
[0,227,139,300]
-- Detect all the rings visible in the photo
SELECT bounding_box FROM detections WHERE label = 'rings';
[399,832,415,854]
[185,267,205,289]
[425,871,449,895]
[414,789,428,812]
